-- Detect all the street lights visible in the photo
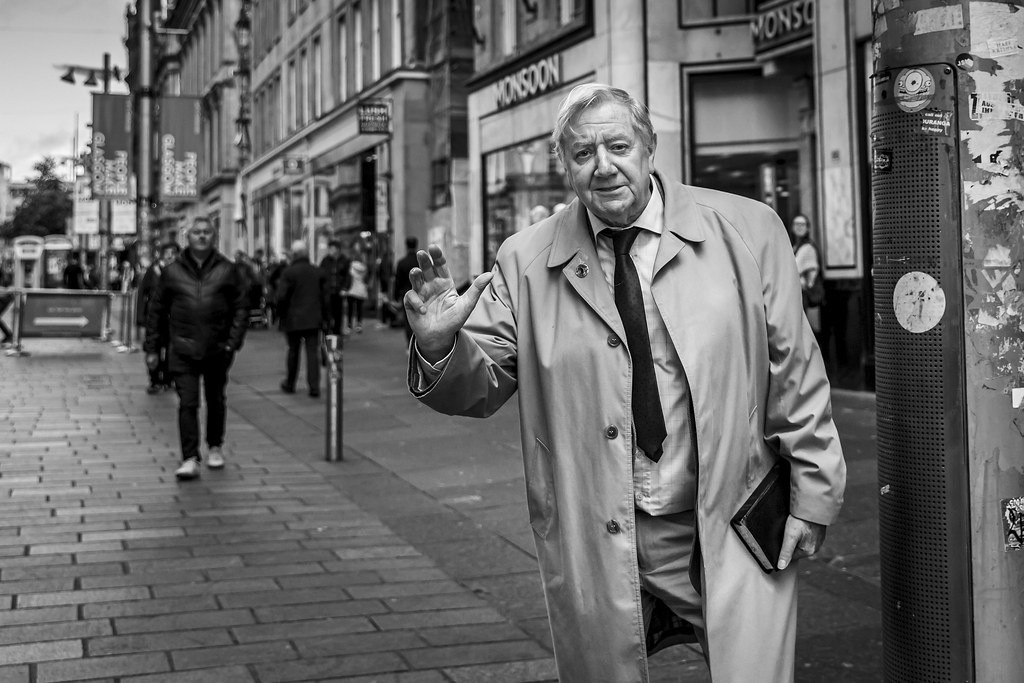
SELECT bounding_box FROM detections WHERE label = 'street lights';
[60,52,127,290]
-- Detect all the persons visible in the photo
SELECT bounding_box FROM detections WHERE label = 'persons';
[789,214,825,337]
[143,216,251,479]
[0,258,16,344]
[62,229,418,399]
[399,83,846,682]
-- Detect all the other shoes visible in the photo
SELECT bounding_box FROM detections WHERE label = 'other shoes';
[175,456,202,480]
[310,394,319,397]
[279,380,295,394]
[147,384,161,394]
[206,447,226,469]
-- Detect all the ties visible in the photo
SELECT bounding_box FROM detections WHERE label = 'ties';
[598,226,668,463]
